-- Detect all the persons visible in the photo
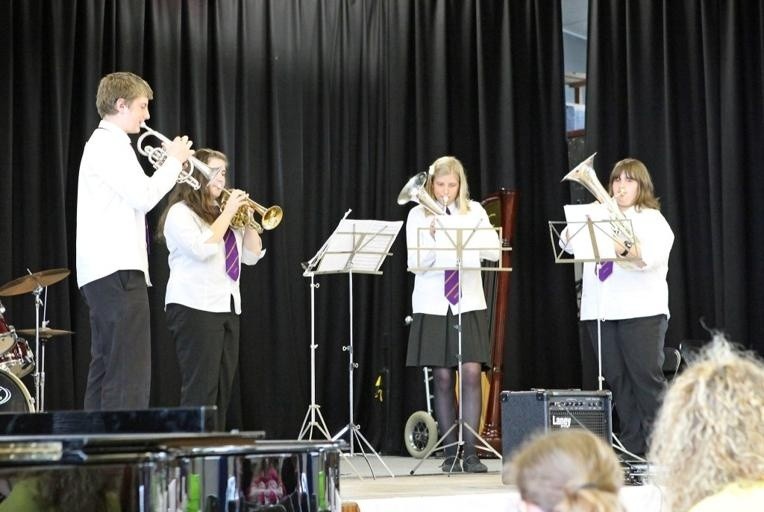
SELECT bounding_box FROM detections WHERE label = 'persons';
[69,70,199,413]
[556,159,675,457]
[513,426,628,512]
[402,153,502,473]
[638,356,763,512]
[156,144,263,435]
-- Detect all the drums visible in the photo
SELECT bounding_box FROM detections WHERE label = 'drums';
[0,338,34,378]
[0,367,34,412]
[0,310,17,354]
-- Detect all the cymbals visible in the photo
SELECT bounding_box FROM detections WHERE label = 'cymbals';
[1,267,67,300]
[18,327,74,338]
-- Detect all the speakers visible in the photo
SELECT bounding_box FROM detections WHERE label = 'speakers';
[502,388,612,466]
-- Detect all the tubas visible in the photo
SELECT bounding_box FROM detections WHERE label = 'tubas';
[563,152,640,261]
[398,172,460,229]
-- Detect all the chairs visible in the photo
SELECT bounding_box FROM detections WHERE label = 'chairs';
[662,347,681,382]
[679,339,711,369]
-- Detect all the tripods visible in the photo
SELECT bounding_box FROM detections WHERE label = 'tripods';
[408,269,501,476]
[579,264,648,463]
[297,276,365,483]
[331,271,395,479]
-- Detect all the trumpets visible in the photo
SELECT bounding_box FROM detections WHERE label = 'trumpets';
[221,189,282,234]
[136,125,219,193]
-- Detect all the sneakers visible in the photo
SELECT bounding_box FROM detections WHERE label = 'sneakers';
[441,453,488,473]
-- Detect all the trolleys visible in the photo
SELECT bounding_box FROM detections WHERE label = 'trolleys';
[402,316,440,459]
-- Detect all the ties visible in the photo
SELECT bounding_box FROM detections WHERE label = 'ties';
[599,261,613,281]
[215,206,239,281]
[444,208,462,305]
